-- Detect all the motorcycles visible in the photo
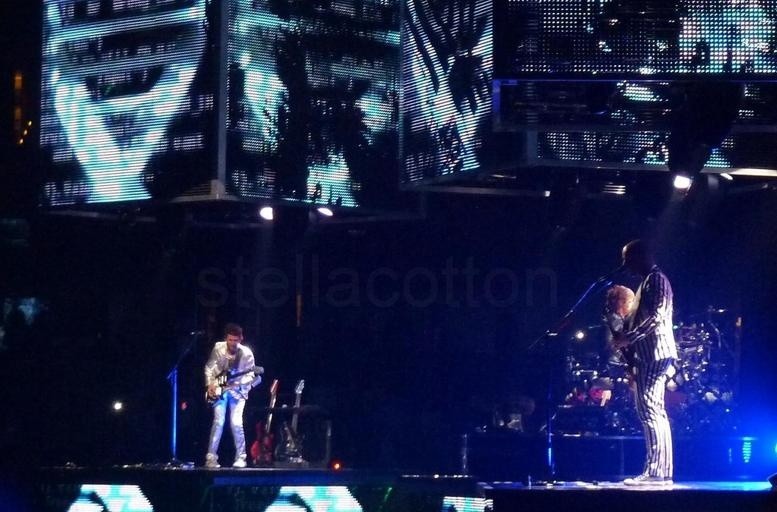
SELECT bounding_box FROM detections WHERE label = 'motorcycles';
[677,352,744,437]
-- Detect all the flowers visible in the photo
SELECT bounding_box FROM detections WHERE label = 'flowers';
[598,265,628,282]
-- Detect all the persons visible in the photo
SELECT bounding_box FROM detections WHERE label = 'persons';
[604,284,636,361]
[204,325,264,469]
[611,236,680,487]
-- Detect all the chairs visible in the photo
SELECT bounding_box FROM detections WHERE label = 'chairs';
[663,475,673,485]
[622,471,664,487]
[231,457,248,467]
[204,457,220,468]
